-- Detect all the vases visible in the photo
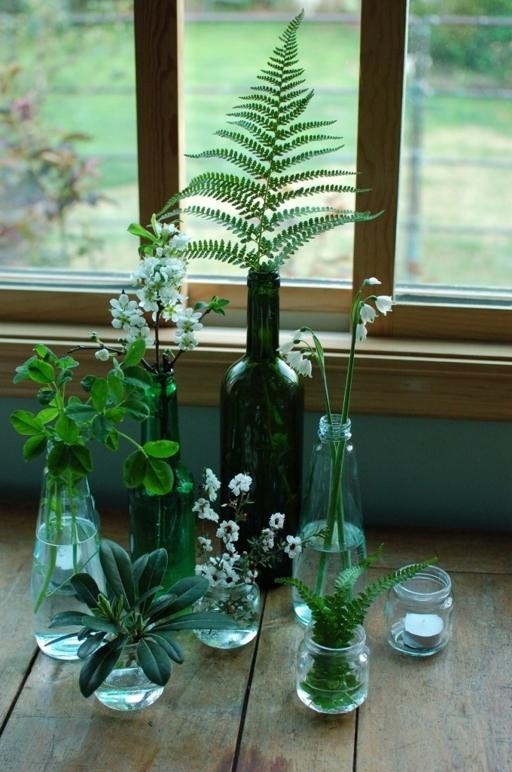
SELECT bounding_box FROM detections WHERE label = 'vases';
[192,554,261,651]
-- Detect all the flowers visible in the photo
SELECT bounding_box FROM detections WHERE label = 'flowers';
[274,276,396,619]
[189,465,302,634]
[65,211,231,583]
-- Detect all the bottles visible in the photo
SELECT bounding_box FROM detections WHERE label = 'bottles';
[90,634,165,711]
[190,568,264,647]
[218,265,305,591]
[29,440,104,661]
[295,620,370,716]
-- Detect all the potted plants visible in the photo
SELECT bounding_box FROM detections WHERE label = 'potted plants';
[144,7,390,588]
[7,342,182,662]
[271,541,440,717]
[46,537,240,712]
